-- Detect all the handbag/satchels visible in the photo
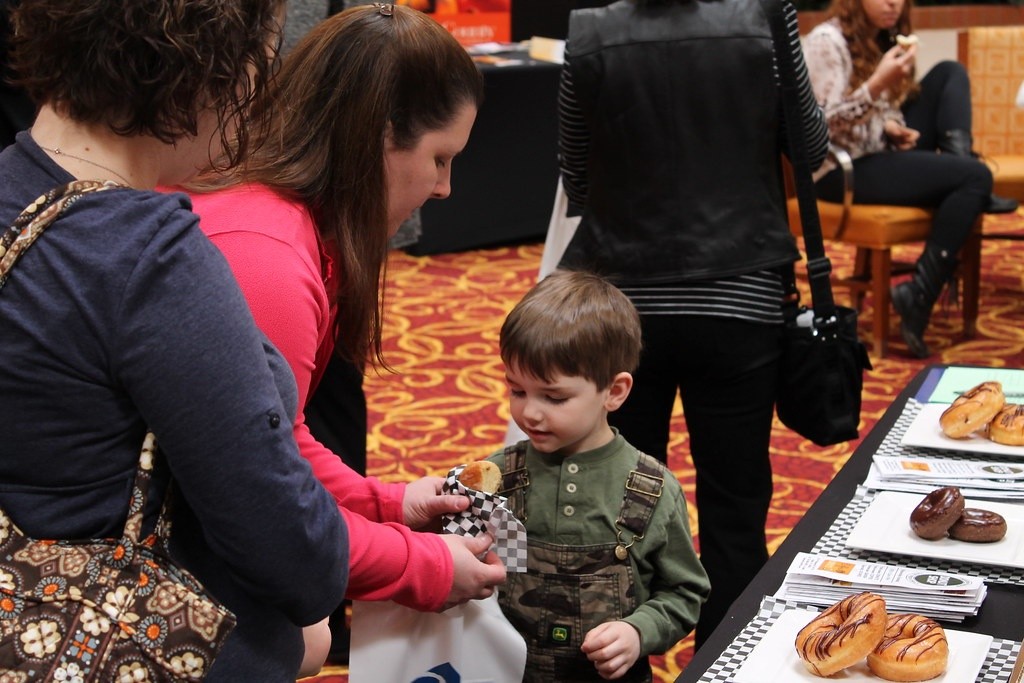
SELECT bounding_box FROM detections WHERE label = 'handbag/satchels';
[775,301,872,447]
[0,178,238,683]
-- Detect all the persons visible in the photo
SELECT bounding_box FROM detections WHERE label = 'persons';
[456,268,712,683]
[799,0,1024,356]
[554,0,827,652]
[154,3,507,683]
[0,1,350,683]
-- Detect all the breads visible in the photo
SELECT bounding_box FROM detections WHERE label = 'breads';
[458,460,502,495]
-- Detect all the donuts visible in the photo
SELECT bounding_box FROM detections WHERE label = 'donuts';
[939,381,1005,439]
[909,487,966,539]
[794,591,888,678]
[896,33,919,47]
[947,508,1007,543]
[985,403,1024,446]
[866,613,949,683]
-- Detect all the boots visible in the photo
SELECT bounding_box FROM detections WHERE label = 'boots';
[939,129,1017,213]
[890,240,962,361]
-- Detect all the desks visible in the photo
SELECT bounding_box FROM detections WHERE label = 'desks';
[392,42,563,253]
[678,364,1024,683]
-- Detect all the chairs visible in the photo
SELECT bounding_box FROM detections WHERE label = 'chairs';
[781,157,984,361]
